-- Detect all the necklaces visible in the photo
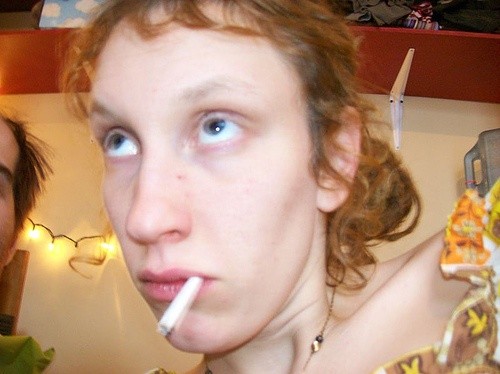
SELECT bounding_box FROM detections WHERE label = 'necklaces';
[202,262,342,374]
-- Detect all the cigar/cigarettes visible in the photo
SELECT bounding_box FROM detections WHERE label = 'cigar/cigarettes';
[155,276,205,336]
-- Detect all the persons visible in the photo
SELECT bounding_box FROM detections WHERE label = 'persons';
[1,110,55,374]
[60,0,500,374]
